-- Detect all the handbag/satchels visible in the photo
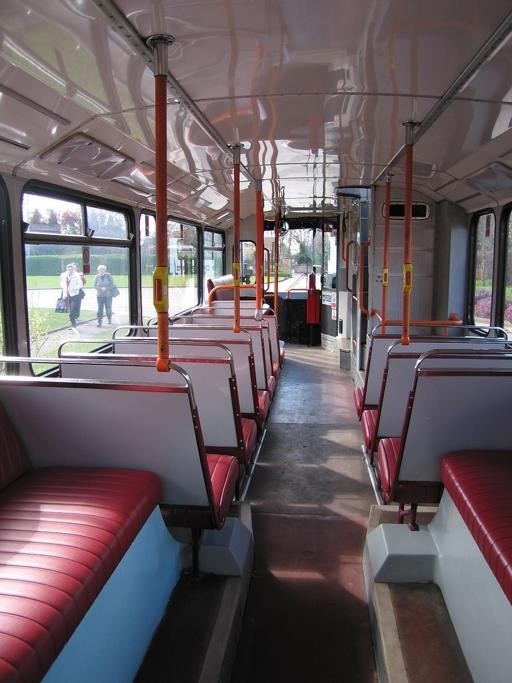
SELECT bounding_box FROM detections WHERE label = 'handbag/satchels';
[55,297,71,314]
[111,287,121,297]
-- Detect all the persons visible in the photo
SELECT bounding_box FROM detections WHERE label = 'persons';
[93,264,120,327]
[55,262,86,331]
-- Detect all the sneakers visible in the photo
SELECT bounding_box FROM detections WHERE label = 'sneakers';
[97,316,112,328]
